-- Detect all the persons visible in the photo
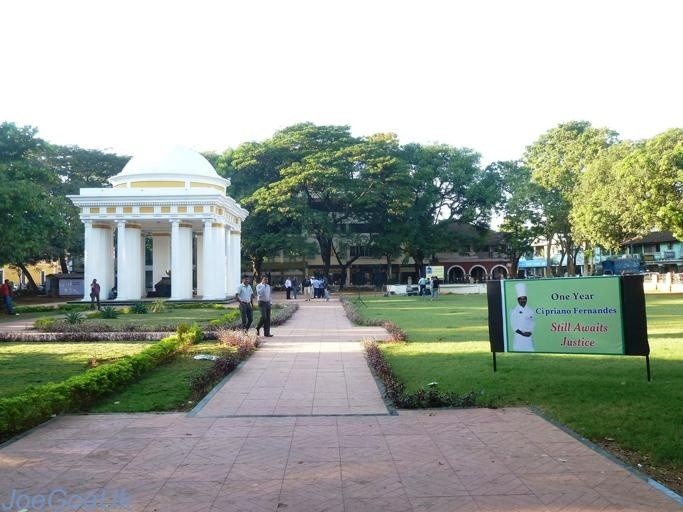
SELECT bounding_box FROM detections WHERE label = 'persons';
[90,279,101,310]
[236,275,254,331]
[255,274,274,337]
[465,274,472,283]
[109,287,117,299]
[0,279,16,315]
[510,296,535,351]
[418,275,440,298]
[284,271,331,302]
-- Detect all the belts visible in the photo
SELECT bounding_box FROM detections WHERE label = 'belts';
[241,302,251,304]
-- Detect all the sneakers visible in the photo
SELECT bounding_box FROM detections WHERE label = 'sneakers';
[243,327,274,337]
[287,296,317,301]
